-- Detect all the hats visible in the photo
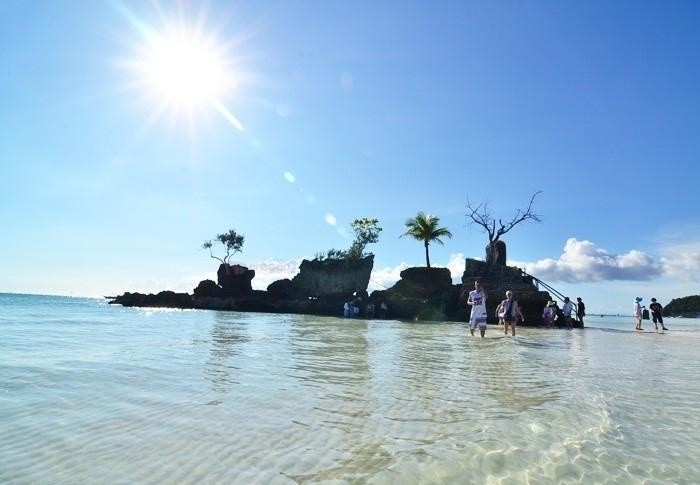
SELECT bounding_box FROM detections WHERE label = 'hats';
[636,296,642,302]
[546,300,555,307]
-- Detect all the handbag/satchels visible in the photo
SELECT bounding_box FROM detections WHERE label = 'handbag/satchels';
[642,310,650,320]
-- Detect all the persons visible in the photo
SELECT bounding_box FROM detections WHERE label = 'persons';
[467,280,525,337]
[343,299,388,320]
[542,297,585,330]
[634,297,669,330]
